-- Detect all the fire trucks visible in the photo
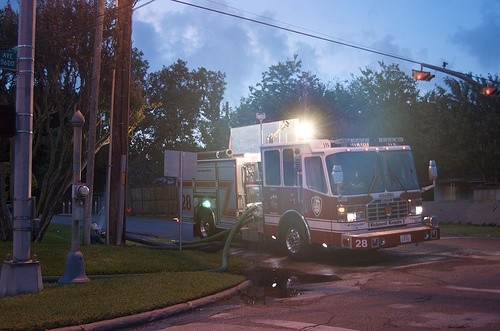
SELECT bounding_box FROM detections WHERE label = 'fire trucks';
[176,120,440,263]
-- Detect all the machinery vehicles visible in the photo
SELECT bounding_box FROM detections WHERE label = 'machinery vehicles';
[249,266,341,298]
